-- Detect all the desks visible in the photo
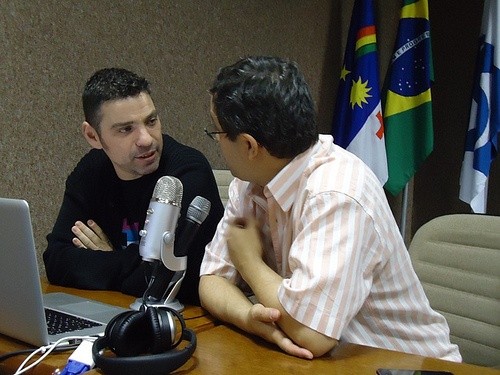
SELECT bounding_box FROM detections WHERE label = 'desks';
[0,281,500,375]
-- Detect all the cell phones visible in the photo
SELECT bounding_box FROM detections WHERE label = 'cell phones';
[377,368,454,375]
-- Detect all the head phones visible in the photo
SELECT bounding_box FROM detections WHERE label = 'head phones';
[91,306,197,375]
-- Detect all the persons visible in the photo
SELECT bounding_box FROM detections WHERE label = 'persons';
[199,56,463,363]
[43,67,224,307]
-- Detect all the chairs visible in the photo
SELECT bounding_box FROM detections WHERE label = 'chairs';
[408,213,500,368]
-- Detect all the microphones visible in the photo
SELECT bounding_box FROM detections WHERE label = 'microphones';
[146,195,211,301]
[130,176,188,314]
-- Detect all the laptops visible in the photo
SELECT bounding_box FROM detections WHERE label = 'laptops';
[0,198,131,352]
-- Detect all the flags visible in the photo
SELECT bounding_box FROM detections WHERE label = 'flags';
[331,0,500,214]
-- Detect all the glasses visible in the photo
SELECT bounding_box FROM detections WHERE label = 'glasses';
[203,118,239,143]
